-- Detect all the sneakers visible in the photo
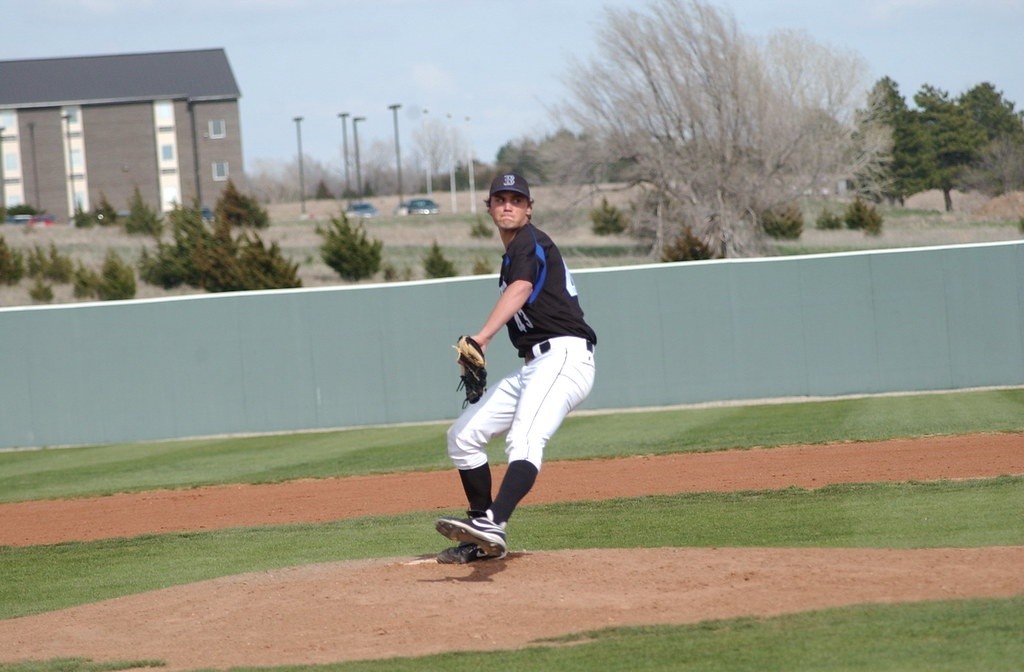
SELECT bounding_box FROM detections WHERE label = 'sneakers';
[437,543,503,562]
[436,514,505,557]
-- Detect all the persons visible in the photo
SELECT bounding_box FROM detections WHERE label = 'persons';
[435,169,597,567]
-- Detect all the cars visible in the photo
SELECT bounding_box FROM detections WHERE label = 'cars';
[393,199,437,217]
[340,203,378,218]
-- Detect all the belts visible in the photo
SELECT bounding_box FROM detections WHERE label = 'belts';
[525,336,595,364]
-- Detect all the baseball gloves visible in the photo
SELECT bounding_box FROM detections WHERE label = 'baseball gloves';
[451,335,487,408]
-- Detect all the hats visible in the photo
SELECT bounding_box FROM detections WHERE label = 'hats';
[490,173,530,199]
